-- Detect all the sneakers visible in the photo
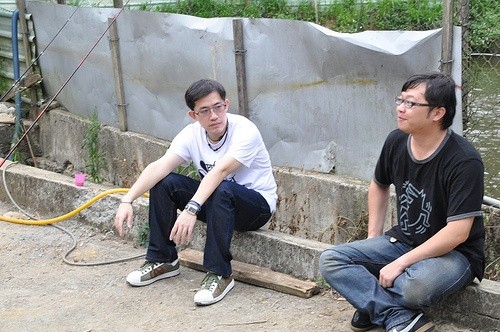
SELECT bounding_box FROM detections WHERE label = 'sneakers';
[387,312,435,332]
[127,258,181,286]
[195,270,234,306]
[351,309,379,332]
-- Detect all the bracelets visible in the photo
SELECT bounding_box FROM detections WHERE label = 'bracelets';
[189,200,202,209]
[188,202,201,212]
[121,201,132,204]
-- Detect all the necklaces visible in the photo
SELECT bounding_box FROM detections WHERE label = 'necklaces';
[206,126,228,141]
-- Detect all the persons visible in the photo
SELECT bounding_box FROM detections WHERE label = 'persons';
[115,79,278,306]
[318,72,485,332]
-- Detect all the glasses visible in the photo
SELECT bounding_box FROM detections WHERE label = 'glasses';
[395,96,446,109]
[194,103,226,117]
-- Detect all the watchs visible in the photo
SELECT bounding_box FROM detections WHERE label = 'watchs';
[185,204,199,215]
